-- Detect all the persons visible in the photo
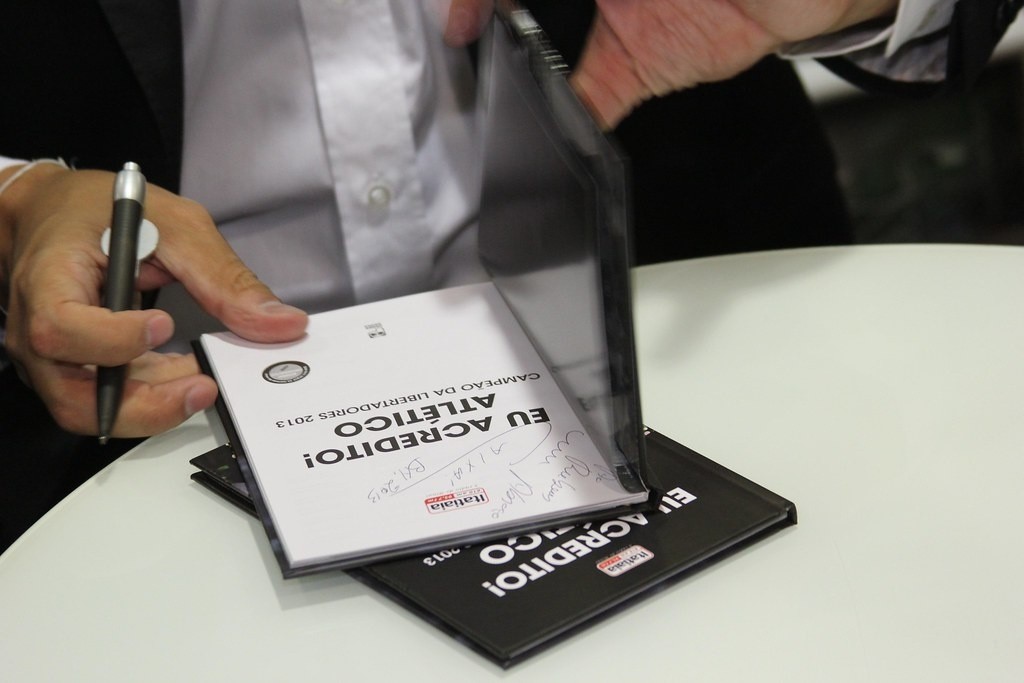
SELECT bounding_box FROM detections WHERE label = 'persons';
[0,0,1024,503]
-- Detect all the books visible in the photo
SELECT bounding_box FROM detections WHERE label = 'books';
[190,424,798,670]
[191,0,663,580]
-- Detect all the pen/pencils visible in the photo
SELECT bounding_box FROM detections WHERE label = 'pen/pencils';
[91,159,162,448]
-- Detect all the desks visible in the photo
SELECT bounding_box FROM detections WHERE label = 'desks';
[0,242,1024,681]
